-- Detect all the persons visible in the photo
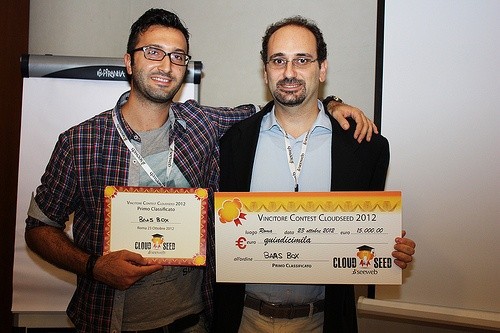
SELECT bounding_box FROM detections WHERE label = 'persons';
[214,16,416,332]
[25,9,379,332]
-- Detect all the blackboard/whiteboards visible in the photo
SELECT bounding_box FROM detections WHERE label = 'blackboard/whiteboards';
[355,0,500,332]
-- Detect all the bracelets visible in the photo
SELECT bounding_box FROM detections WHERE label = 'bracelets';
[86,254,99,280]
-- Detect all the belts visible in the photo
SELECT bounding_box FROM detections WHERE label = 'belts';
[121,309,208,333]
[244,294,325,319]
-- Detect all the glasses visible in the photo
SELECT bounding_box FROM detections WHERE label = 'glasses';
[265,57,319,69]
[130,46,192,66]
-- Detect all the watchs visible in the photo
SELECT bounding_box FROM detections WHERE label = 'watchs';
[322,95,343,115]
